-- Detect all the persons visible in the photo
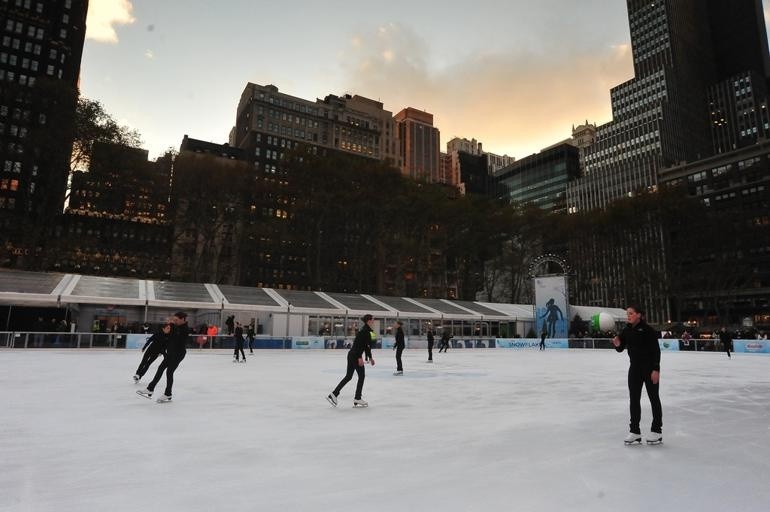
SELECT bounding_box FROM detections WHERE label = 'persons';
[427,331,434,361]
[136,325,170,379]
[394,321,405,371]
[138,312,191,401]
[539,299,563,338]
[329,314,375,405]
[33,317,44,346]
[612,304,663,441]
[572,327,769,356]
[439,333,453,352]
[109,315,259,363]
[540,330,547,350]
[47,318,56,345]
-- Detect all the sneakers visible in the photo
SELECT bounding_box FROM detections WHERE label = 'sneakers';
[354,399,368,405]
[625,432,641,441]
[158,395,171,400]
[647,432,662,441]
[329,393,339,406]
[140,389,153,397]
